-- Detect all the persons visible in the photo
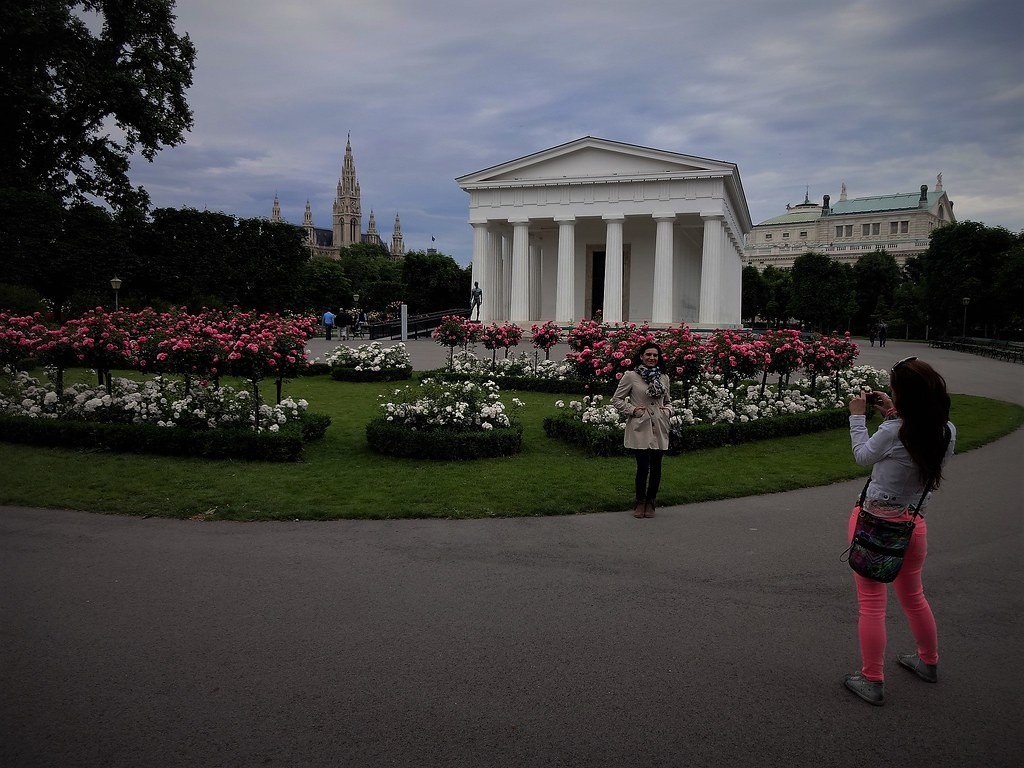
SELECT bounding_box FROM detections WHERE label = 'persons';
[868,318,889,347]
[613,342,674,518]
[842,354,957,706]
[323,307,367,340]
[468,281,483,321]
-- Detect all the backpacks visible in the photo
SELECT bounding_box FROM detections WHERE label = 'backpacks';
[881,327,886,336]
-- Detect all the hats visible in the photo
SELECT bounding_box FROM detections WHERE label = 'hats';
[340,308,343,311]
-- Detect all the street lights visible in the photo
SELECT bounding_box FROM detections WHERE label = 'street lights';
[110,275,122,312]
[962,297,972,336]
[352,292,360,321]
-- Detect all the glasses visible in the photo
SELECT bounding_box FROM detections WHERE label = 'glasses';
[891,356,918,376]
[644,353,658,357]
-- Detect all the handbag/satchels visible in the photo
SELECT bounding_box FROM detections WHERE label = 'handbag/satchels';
[840,510,916,583]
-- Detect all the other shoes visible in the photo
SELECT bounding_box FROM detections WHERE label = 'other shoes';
[343,335,345,341]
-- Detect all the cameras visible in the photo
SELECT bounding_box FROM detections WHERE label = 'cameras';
[865,393,877,404]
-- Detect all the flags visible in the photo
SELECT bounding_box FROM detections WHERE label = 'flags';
[432,236,435,242]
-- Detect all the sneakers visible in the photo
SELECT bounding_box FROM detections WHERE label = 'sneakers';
[897,650,940,683]
[635,504,655,518]
[844,671,885,706]
